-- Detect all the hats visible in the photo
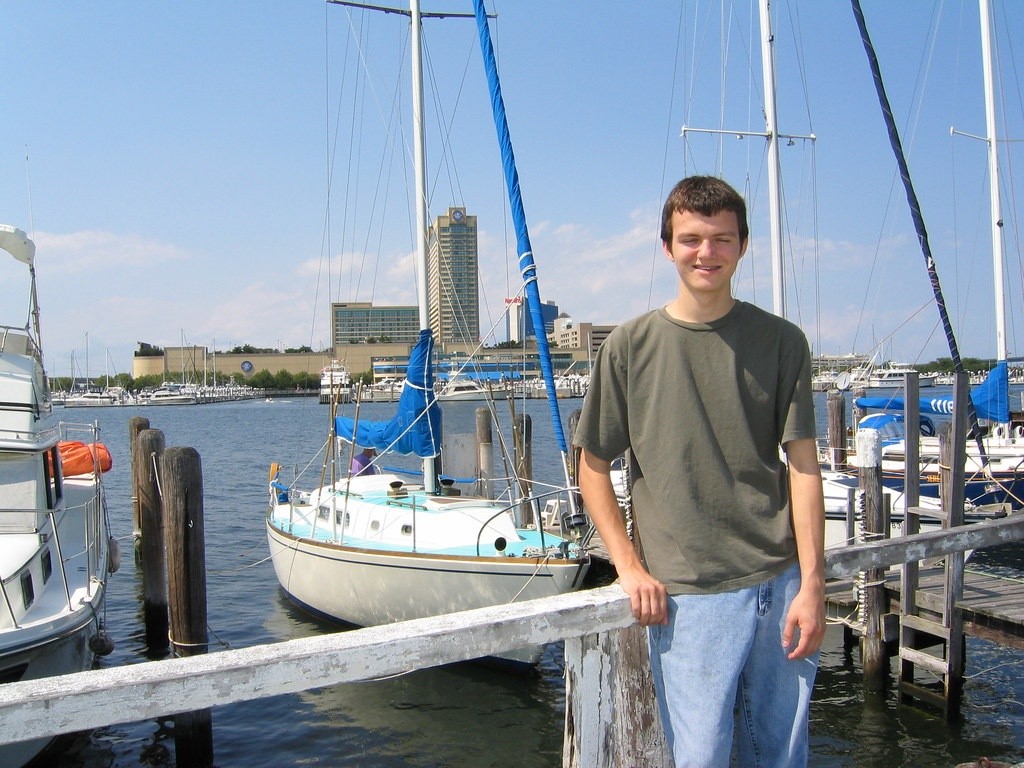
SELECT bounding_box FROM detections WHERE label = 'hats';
[365,449,378,457]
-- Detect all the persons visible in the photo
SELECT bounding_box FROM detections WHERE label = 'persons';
[571,176,826,768]
[350,447,378,476]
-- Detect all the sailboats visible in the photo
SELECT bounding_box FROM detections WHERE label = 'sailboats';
[316,0,1022,543]
[44,330,274,408]
[265,2,602,680]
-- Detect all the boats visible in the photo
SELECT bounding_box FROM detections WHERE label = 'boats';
[0,225,121,768]
[317,347,355,405]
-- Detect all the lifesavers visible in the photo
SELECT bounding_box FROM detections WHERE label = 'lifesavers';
[919,415,936,436]
